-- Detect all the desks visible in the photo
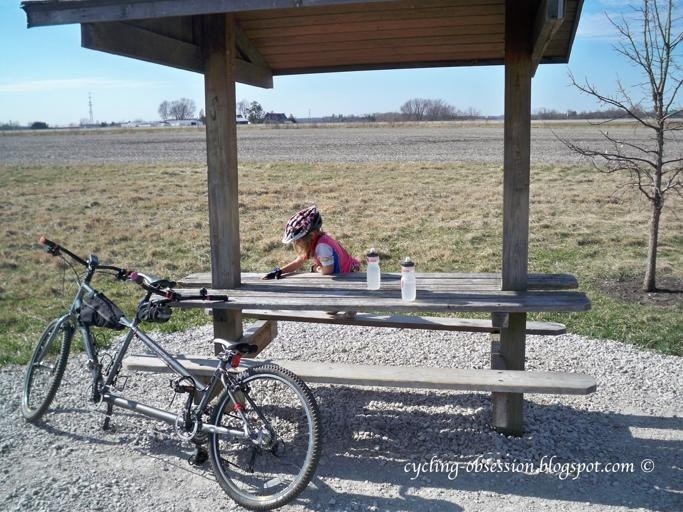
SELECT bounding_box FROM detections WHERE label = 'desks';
[138,269,591,438]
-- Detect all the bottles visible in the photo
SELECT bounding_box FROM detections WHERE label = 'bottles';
[400,257,416,302]
[367,248,380,290]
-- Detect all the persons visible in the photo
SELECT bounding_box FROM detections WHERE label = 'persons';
[262,205,360,318]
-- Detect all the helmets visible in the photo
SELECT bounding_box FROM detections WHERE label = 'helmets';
[281,205,319,245]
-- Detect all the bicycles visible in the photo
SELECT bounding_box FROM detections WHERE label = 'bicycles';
[21,236,322,512]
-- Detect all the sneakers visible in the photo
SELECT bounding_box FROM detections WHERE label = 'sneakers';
[327,310,357,319]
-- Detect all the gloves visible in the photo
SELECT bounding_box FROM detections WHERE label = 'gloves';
[263,267,282,280]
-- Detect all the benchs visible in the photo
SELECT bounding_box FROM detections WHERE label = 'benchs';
[125,309,597,438]
